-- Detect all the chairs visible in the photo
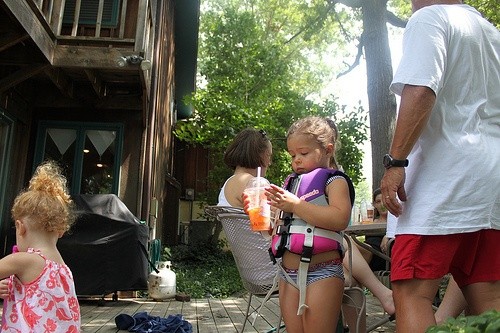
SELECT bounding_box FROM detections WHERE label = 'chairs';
[206,205,368,333]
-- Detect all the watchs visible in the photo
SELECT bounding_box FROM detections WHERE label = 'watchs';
[383,154,409,169]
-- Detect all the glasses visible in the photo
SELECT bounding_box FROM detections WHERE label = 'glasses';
[259,128,266,139]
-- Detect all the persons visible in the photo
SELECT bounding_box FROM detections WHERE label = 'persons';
[380,2,500,333]
[241,116,355,333]
[218,129,396,333]
[0,159,84,333]
[357,188,390,270]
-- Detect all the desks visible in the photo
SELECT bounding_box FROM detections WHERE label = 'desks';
[346,222,439,333]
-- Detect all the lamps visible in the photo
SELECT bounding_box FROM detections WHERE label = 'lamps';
[108,49,151,70]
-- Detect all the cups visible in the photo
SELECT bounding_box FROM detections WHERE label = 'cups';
[244,177,271,231]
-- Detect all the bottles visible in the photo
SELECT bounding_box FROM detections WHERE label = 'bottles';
[354,201,361,222]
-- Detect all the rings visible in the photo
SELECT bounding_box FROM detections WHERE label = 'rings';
[385,196,389,199]
